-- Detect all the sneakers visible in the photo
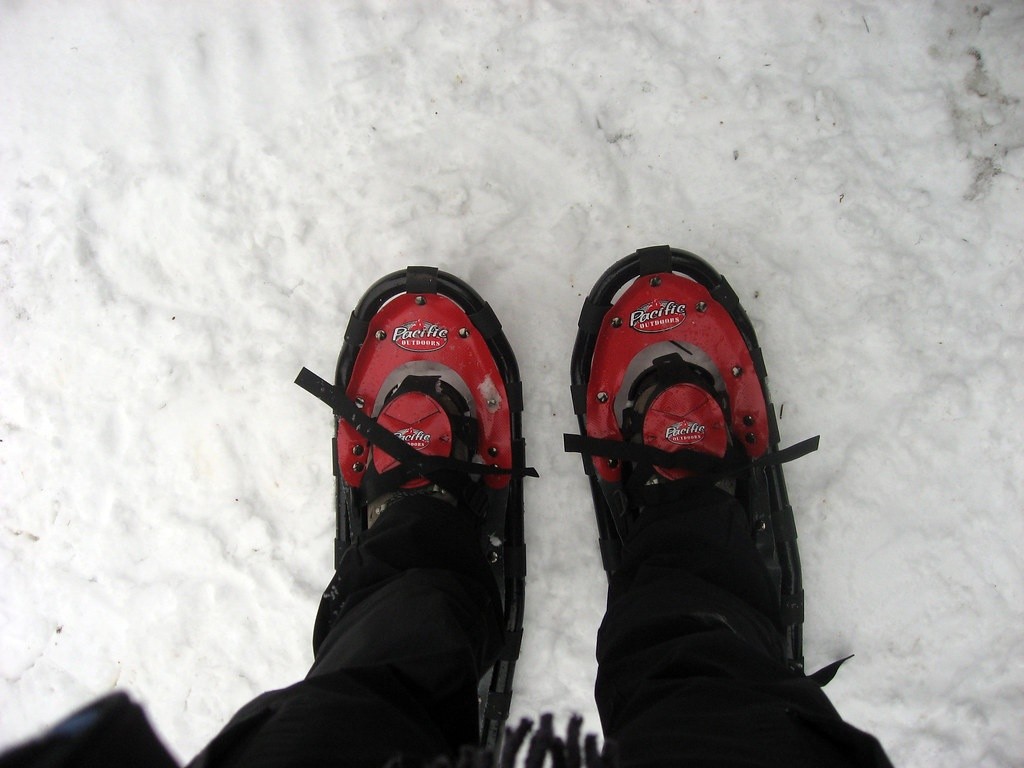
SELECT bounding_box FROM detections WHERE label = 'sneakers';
[613,340,739,526]
[365,360,479,542]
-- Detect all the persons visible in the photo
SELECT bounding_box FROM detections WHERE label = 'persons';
[1,246,893,768]
